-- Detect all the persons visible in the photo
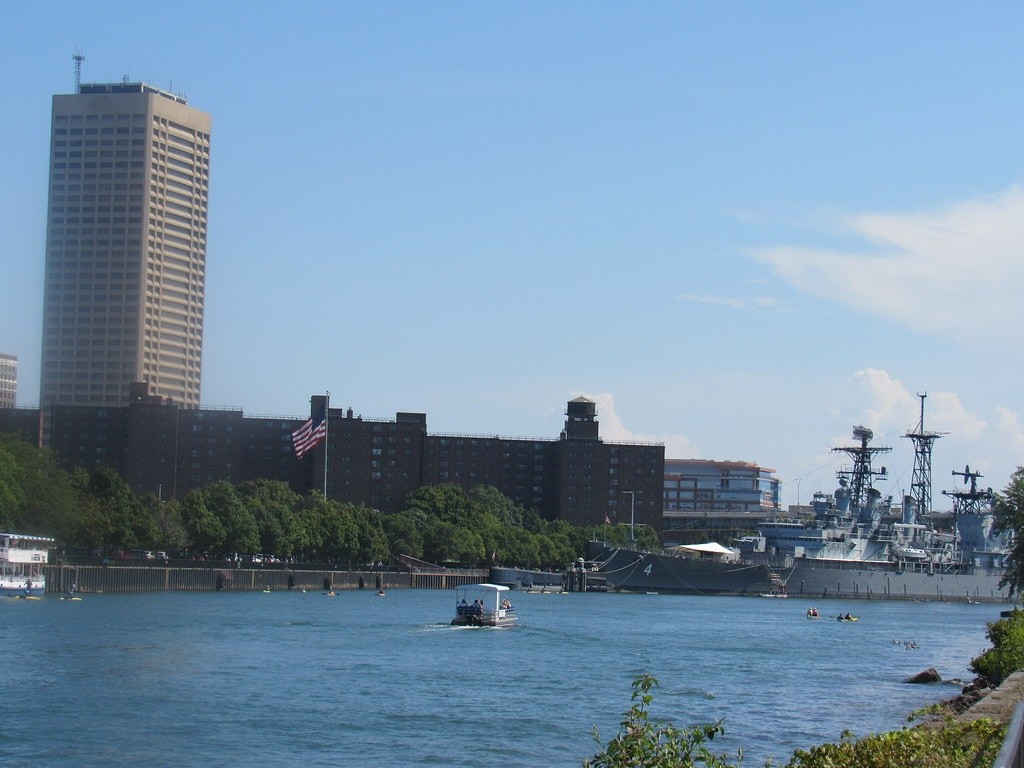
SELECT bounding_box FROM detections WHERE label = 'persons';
[118,551,769,598]
[0,540,76,600]
[807,607,852,621]
[458,597,512,621]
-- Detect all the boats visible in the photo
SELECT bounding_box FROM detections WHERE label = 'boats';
[451,583,521,628]
[839,616,860,623]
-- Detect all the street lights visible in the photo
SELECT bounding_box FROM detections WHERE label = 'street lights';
[622,490,635,539]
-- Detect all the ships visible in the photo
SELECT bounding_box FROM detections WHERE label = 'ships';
[488,391,1024,601]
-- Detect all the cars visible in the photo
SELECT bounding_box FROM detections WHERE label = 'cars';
[143,550,155,560]
[226,552,280,565]
[157,552,169,560]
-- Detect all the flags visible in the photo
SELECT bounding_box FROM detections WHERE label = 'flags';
[292,401,326,461]
